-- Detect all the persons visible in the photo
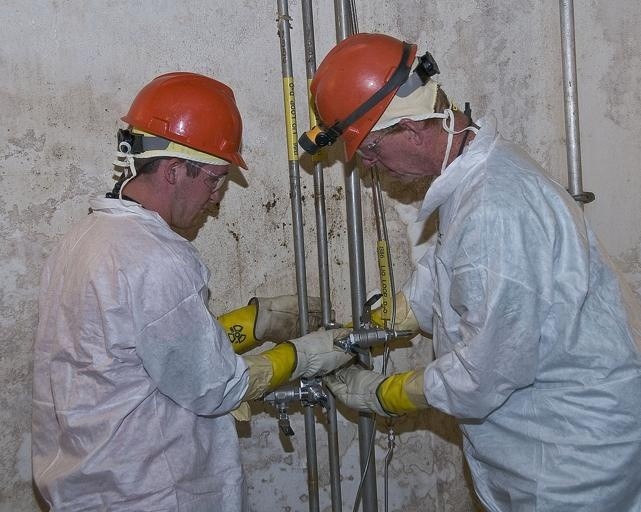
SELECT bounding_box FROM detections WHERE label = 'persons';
[24,70,357,511]
[300,25,641,511]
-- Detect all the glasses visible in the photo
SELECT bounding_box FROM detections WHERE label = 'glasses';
[356,119,408,162]
[179,157,229,194]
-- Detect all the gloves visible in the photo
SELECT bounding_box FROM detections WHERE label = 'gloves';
[321,361,407,427]
[250,291,339,345]
[288,325,357,385]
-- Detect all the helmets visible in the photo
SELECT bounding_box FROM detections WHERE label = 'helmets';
[308,31,421,164]
[117,70,254,172]
